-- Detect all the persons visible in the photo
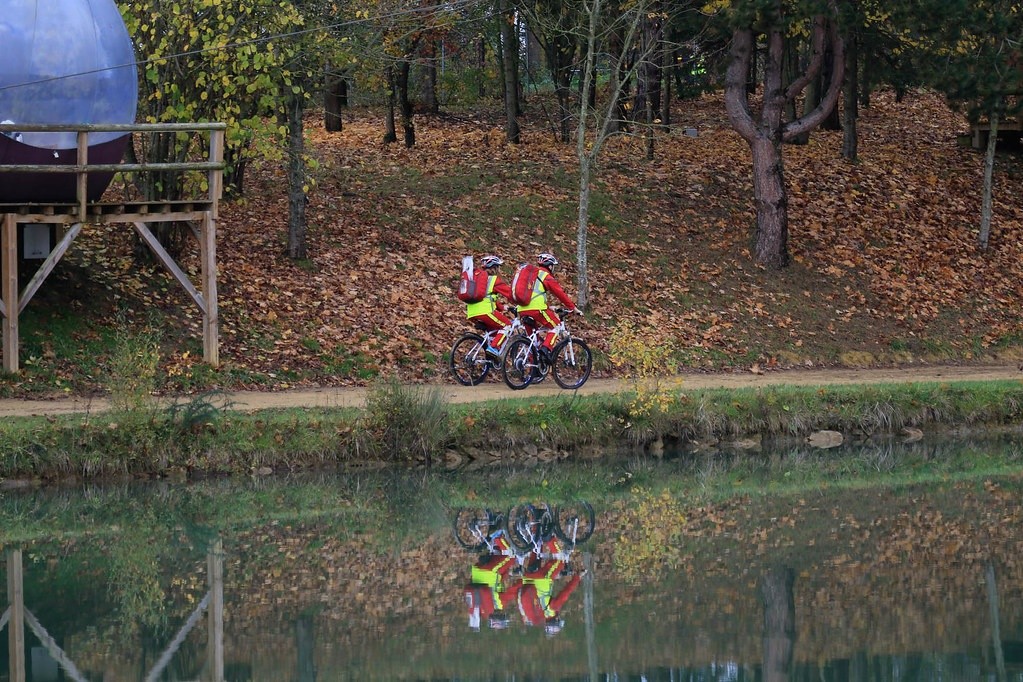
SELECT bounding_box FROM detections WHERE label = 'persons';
[467,256,515,373]
[472,531,588,640]
[517,254,583,378]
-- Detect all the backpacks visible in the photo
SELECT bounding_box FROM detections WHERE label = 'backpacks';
[458,267,497,303]
[511,264,546,306]
[464,584,502,620]
[516,584,552,628]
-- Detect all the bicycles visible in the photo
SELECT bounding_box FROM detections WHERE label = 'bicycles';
[449,306,550,387]
[501,305,592,389]
[454,502,595,571]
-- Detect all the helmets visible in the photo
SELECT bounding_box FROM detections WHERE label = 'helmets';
[537,253,558,265]
[489,619,510,630]
[481,255,502,268]
[544,625,561,636]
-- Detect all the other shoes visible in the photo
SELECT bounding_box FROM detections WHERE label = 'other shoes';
[489,530,503,538]
[538,346,553,365]
[485,345,499,357]
[542,523,556,541]
[529,367,543,377]
